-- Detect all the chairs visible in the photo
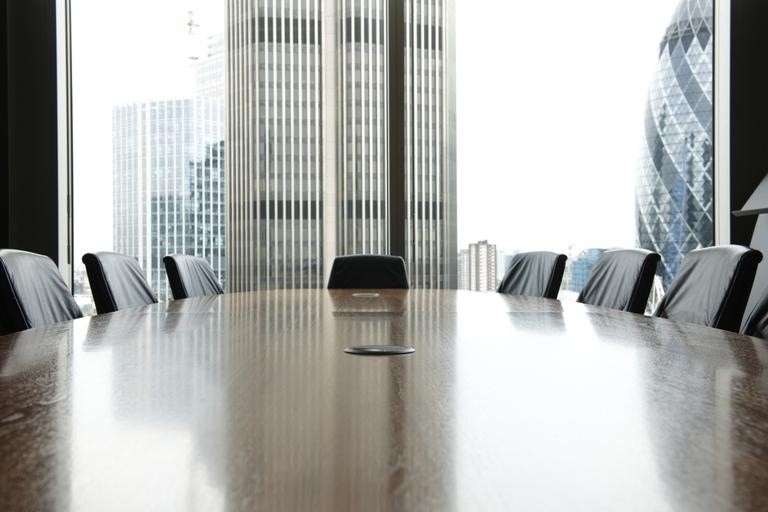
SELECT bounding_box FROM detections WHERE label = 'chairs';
[497,244,764,333]
[0,246,226,331]
[327,254,411,290]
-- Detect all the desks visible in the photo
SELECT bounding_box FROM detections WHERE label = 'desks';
[0,288,766,512]
